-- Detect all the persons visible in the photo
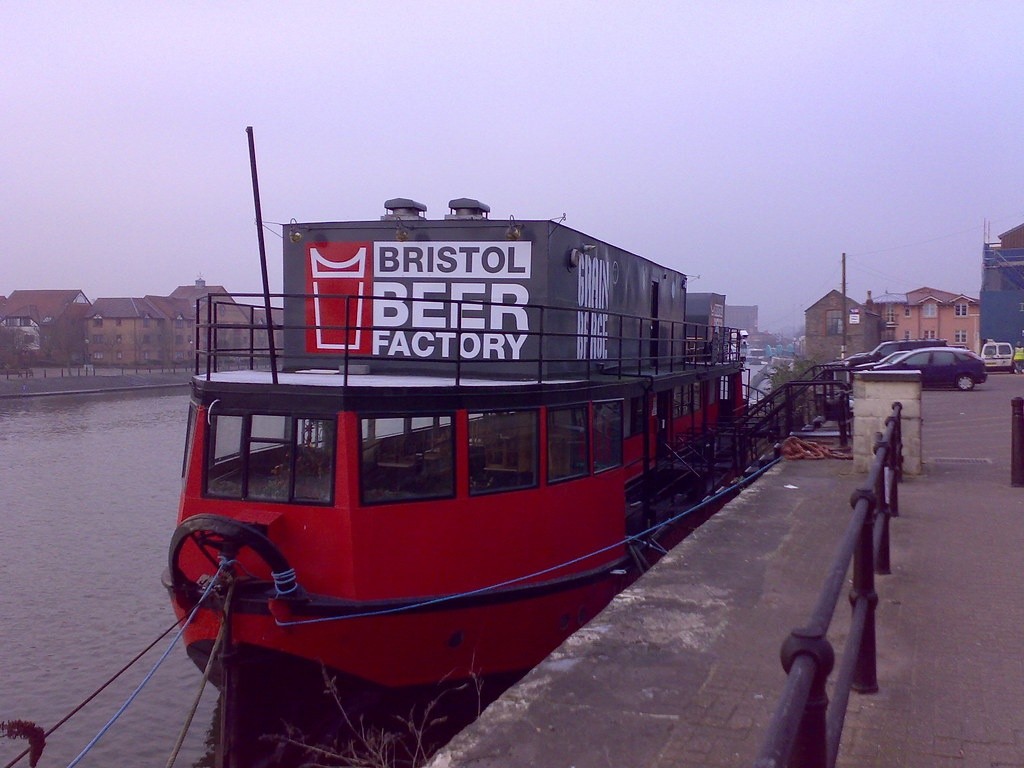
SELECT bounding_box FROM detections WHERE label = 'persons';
[1012,341,1024,375]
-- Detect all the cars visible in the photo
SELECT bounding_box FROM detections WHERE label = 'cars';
[852,350,911,373]
[871,348,989,391]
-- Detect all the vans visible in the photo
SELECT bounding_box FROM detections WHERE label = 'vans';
[981,342,1014,371]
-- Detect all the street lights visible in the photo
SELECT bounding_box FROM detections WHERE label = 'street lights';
[85,339,89,375]
[190,340,193,371]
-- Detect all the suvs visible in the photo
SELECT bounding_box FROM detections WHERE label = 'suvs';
[844,338,951,372]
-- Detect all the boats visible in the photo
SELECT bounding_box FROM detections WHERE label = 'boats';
[158,125,751,702]
